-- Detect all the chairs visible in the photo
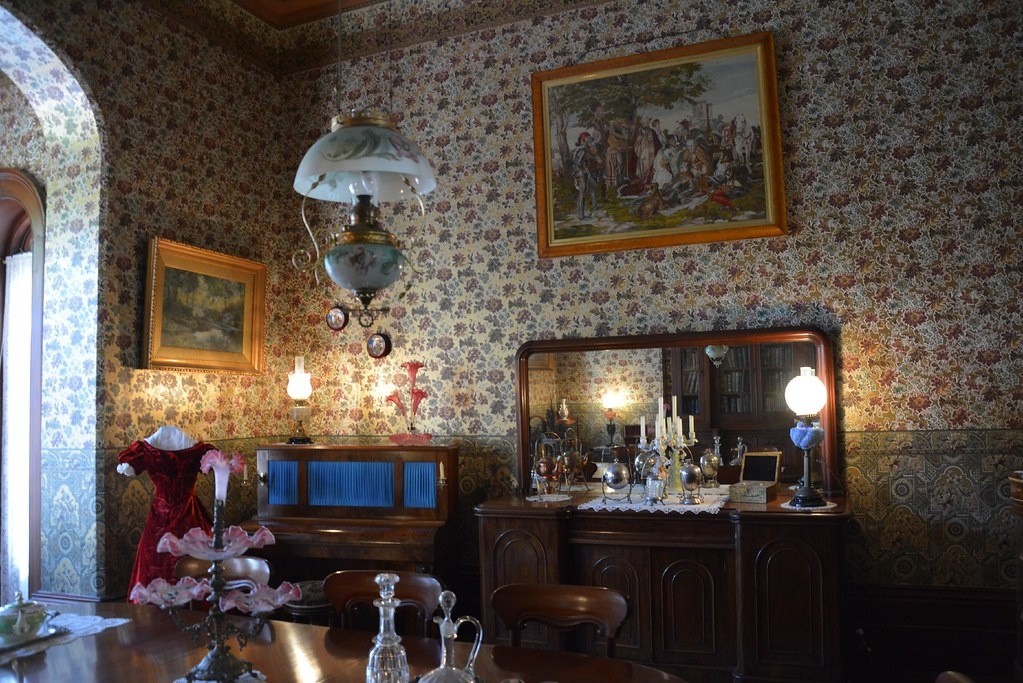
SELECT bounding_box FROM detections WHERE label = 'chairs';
[490,584,633,656]
[173,553,245,612]
[322,569,443,638]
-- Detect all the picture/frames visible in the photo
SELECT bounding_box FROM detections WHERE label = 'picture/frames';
[530,33,788,257]
[142,235,268,376]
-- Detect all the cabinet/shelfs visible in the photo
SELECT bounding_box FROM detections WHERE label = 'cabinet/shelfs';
[474,498,854,683]
[589,448,641,462]
[672,343,818,475]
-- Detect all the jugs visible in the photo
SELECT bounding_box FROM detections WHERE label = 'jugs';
[417,591,483,683]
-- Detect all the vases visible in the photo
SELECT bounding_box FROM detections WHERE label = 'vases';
[559,398,569,420]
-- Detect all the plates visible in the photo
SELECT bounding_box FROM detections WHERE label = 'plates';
[285,579,332,611]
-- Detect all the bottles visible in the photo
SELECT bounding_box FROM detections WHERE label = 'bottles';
[365,572,411,683]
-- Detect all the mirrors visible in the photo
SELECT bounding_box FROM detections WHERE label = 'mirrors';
[516,327,837,505]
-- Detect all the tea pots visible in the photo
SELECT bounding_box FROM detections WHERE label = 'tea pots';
[534,433,563,475]
[559,438,590,473]
[0,588,48,641]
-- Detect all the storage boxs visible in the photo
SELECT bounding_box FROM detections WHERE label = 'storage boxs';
[729,452,782,504]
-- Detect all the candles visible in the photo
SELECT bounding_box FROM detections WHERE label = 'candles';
[641,417,646,439]
[689,415,694,432]
[655,396,683,439]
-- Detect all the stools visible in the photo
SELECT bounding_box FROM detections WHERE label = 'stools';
[282,580,331,627]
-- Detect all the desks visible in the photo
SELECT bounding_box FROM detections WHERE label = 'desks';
[0,604,687,683]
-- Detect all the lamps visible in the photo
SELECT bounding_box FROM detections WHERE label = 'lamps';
[600,408,618,447]
[784,367,828,509]
[285,356,313,445]
[705,344,729,368]
[384,359,432,444]
[292,0,437,315]
